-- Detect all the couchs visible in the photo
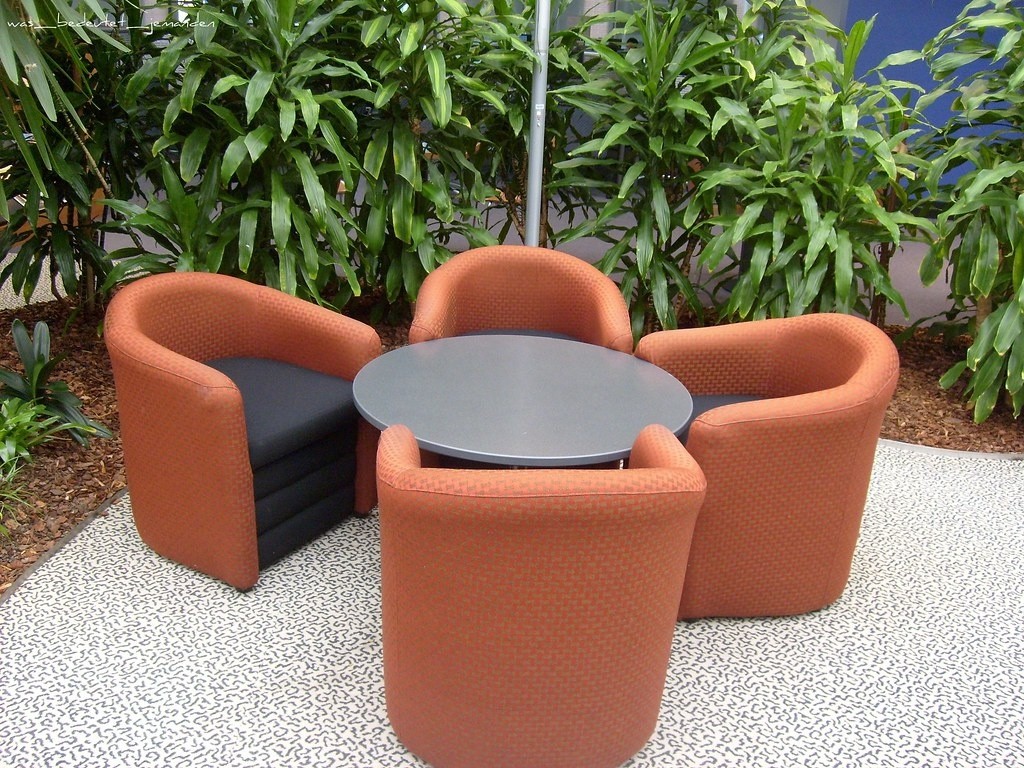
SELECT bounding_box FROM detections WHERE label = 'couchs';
[103,272,383,594]
[408,243,634,469]
[622,312,900,624]
[375,422,708,768]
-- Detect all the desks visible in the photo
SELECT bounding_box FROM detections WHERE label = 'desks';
[352,335,694,469]
